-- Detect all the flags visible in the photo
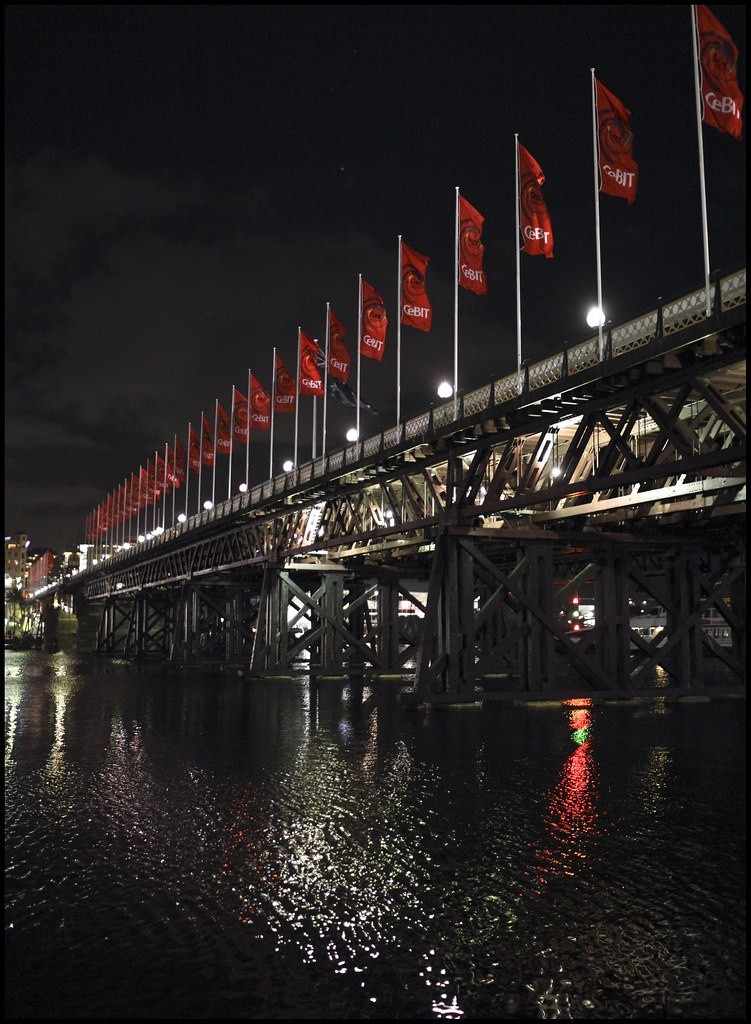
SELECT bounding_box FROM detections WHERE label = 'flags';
[275,353,296,413]
[233,390,248,443]
[189,426,199,471]
[299,331,325,395]
[175,438,185,479]
[518,142,554,258]
[360,282,387,361]
[329,310,350,383]
[594,76,638,204]
[401,242,432,333]
[459,195,486,296]
[202,417,213,465]
[697,3,744,140]
[86,445,180,538]
[29,551,53,582]
[249,374,270,429]
[217,404,230,453]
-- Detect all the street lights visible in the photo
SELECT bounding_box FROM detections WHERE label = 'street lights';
[283,460,294,491]
[239,483,248,509]
[437,381,453,425]
[204,501,213,523]
[586,307,606,362]
[346,428,359,462]
[177,513,186,535]
